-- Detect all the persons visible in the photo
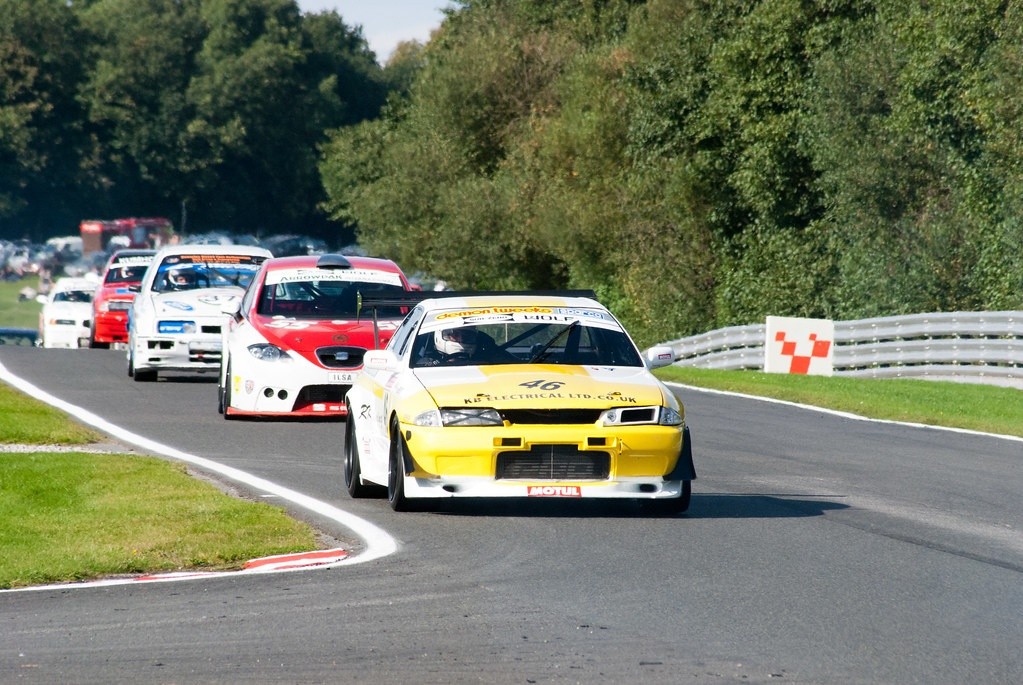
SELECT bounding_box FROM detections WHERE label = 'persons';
[418,325,478,363]
[166,270,197,290]
[119,265,142,281]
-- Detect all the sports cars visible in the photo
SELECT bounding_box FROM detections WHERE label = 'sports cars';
[219,254,419,418]
[127,244,274,383]
[342,290,697,517]
[89,248,161,350]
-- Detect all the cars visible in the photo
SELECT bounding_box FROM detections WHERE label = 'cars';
[0,220,368,350]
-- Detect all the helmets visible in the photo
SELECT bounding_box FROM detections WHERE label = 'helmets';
[120,267,135,278]
[168,269,197,290]
[434,326,478,355]
